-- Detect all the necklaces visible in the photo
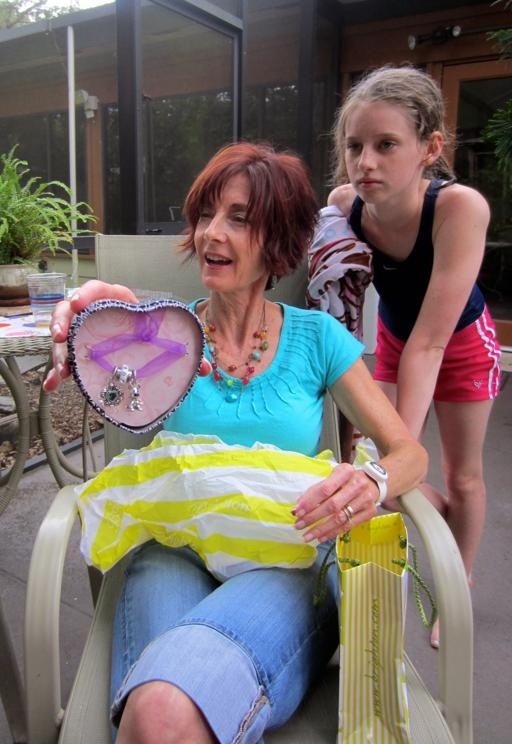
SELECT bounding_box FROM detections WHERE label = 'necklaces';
[197,301,280,404]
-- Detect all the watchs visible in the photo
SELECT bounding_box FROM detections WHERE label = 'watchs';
[355,455,389,512]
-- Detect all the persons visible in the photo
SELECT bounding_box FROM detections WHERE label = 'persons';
[42,141,428,744]
[313,64,503,649]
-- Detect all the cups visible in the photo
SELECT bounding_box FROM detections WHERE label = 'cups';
[26,272,68,328]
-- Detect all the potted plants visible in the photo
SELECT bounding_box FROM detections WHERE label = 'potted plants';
[0,135,108,308]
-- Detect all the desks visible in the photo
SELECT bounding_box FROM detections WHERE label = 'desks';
[1,282,192,523]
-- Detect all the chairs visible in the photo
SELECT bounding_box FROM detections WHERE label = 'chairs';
[17,382,484,744]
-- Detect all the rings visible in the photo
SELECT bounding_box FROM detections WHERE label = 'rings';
[338,500,359,524]
